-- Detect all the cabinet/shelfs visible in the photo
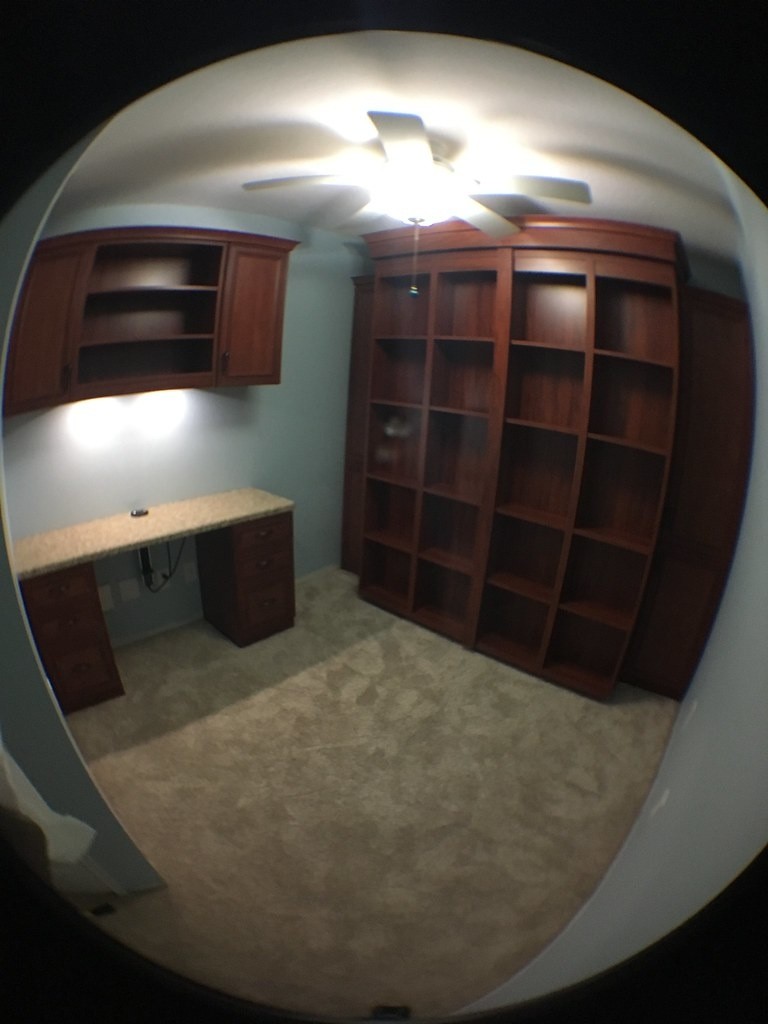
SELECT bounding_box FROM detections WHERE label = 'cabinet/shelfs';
[338,214,694,709]
[3,224,302,417]
[195,510,296,648]
[19,562,127,716]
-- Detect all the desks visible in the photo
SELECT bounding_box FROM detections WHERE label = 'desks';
[17,488,295,582]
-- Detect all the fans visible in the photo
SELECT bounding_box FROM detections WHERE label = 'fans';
[242,102,594,297]
